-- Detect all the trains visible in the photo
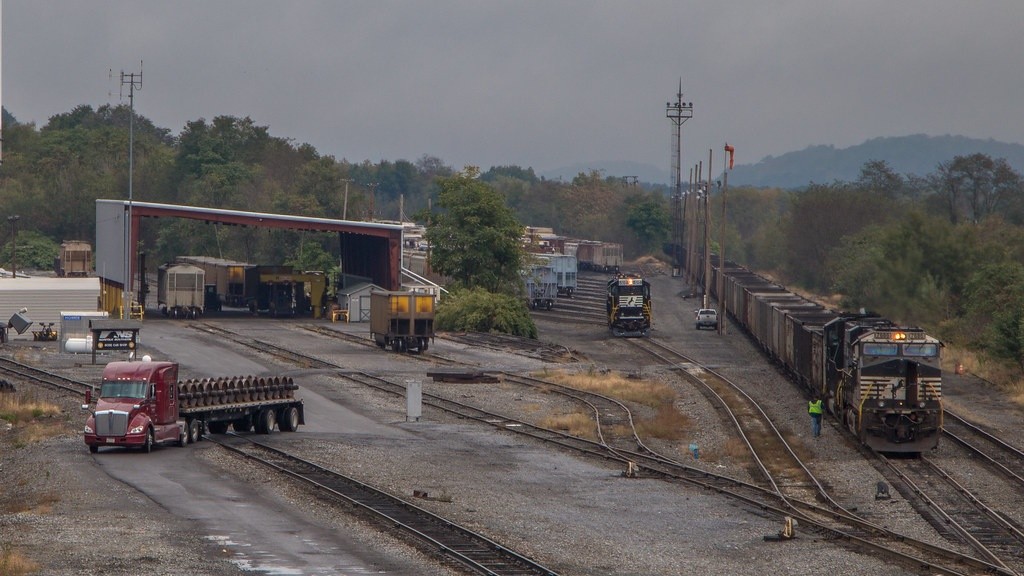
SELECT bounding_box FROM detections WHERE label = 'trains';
[605,263,655,339]
[658,242,944,457]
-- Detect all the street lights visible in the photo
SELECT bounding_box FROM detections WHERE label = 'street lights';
[338,178,355,220]
[7,215,21,278]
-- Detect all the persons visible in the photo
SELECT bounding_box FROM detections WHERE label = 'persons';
[807,393,825,439]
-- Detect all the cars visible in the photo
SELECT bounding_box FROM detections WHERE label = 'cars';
[694,308,718,329]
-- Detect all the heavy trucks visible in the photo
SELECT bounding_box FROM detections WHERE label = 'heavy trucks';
[83,356,305,453]
